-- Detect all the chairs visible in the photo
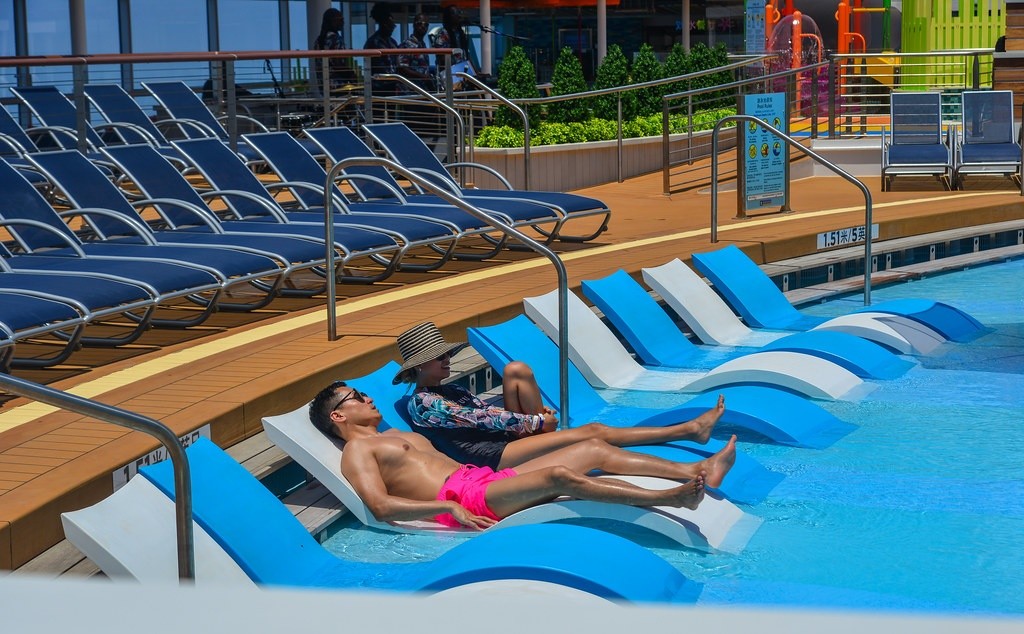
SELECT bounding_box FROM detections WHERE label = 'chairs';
[465,313,860,449]
[641,257,958,359]
[581,269,916,380]
[952,89,1024,194]
[692,244,997,343]
[0,81,613,373]
[260,397,765,556]
[522,287,880,404]
[61,474,259,590]
[138,435,705,607]
[334,359,787,507]
[881,89,952,191]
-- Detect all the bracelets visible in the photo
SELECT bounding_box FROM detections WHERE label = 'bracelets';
[538,412,545,430]
[532,413,538,431]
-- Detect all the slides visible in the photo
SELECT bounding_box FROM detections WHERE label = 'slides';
[855,51,901,92]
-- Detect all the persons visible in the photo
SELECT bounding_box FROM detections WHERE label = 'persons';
[313,8,357,97]
[309,380,739,531]
[392,321,726,474]
[434,5,481,76]
[399,13,446,92]
[363,2,404,98]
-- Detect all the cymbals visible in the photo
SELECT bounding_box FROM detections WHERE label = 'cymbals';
[330,84,364,92]
[271,79,323,88]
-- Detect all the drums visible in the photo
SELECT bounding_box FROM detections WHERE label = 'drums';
[280,113,325,138]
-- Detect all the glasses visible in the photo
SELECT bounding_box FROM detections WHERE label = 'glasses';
[335,17,345,20]
[332,388,368,422]
[434,349,451,361]
[414,21,429,27]
[449,10,464,14]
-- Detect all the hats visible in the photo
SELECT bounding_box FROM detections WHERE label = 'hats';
[391,321,469,386]
